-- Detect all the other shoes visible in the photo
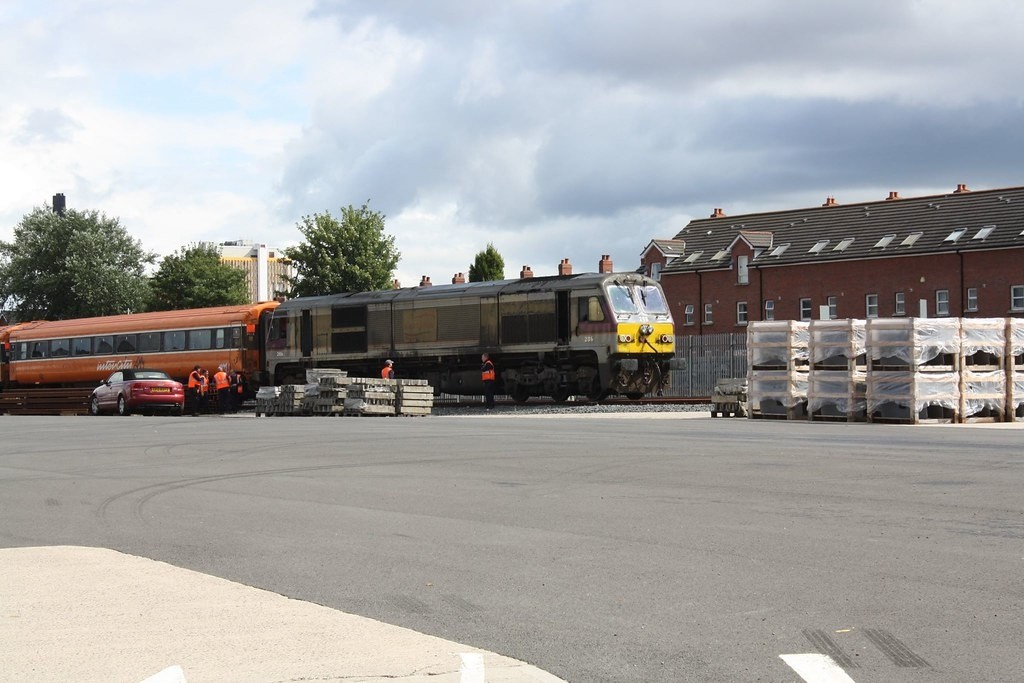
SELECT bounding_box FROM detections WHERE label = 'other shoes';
[192,413,200,416]
[484,403,494,409]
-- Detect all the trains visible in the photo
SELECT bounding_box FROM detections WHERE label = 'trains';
[0,268,676,405]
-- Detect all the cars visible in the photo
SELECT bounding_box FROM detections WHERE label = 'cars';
[89,368,185,416]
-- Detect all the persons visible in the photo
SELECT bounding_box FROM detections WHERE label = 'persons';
[481,353,495,410]
[381,360,394,379]
[187,365,242,418]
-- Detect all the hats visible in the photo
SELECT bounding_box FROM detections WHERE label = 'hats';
[230,370,234,372]
[217,367,223,371]
[385,359,394,365]
